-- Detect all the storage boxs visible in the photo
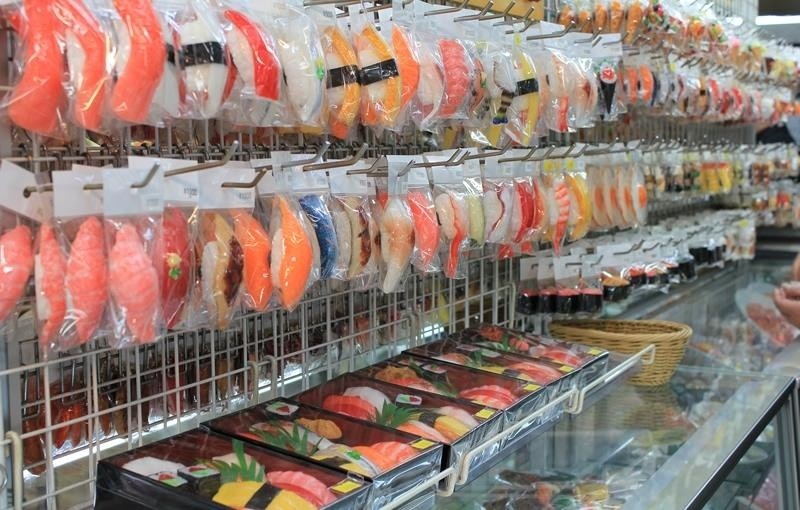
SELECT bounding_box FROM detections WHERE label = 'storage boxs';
[400,336,582,428]
[199,395,447,510]
[288,371,509,492]
[450,323,611,391]
[93,425,370,510]
[350,352,553,457]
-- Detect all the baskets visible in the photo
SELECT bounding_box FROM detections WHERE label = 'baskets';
[546,317,695,387]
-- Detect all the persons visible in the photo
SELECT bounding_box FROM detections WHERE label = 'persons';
[772,256,799,327]
